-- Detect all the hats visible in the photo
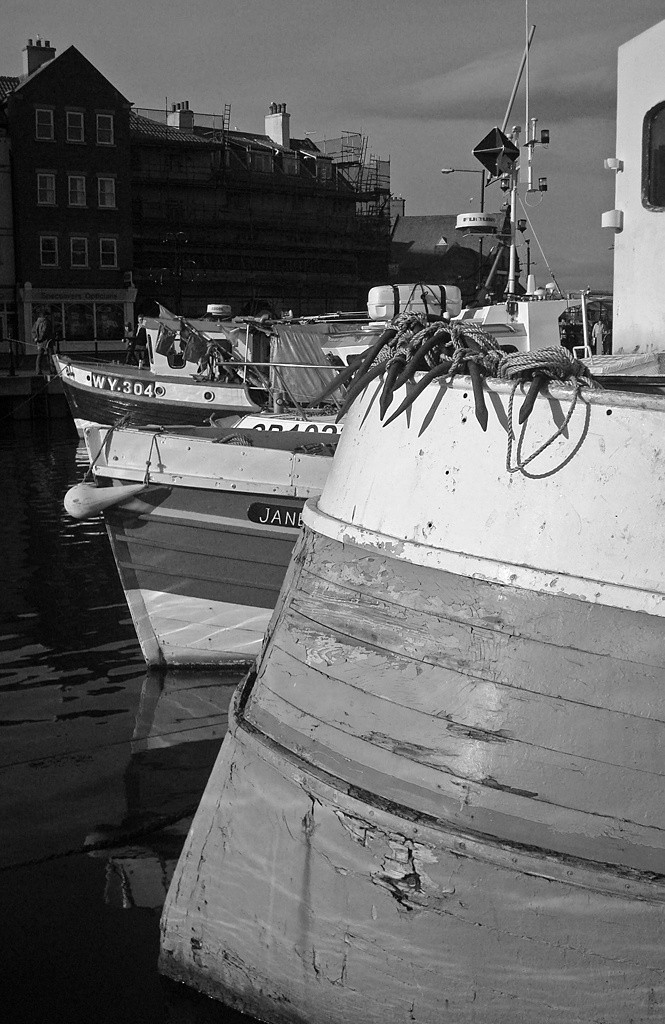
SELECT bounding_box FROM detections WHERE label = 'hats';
[41,312,50,315]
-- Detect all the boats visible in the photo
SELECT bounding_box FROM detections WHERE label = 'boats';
[153,12,665,1024]
[48,0,611,667]
[123,663,251,843]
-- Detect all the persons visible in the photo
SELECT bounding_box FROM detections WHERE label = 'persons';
[591,315,609,356]
[559,317,576,346]
[133,313,150,370]
[31,309,59,375]
[122,321,140,366]
